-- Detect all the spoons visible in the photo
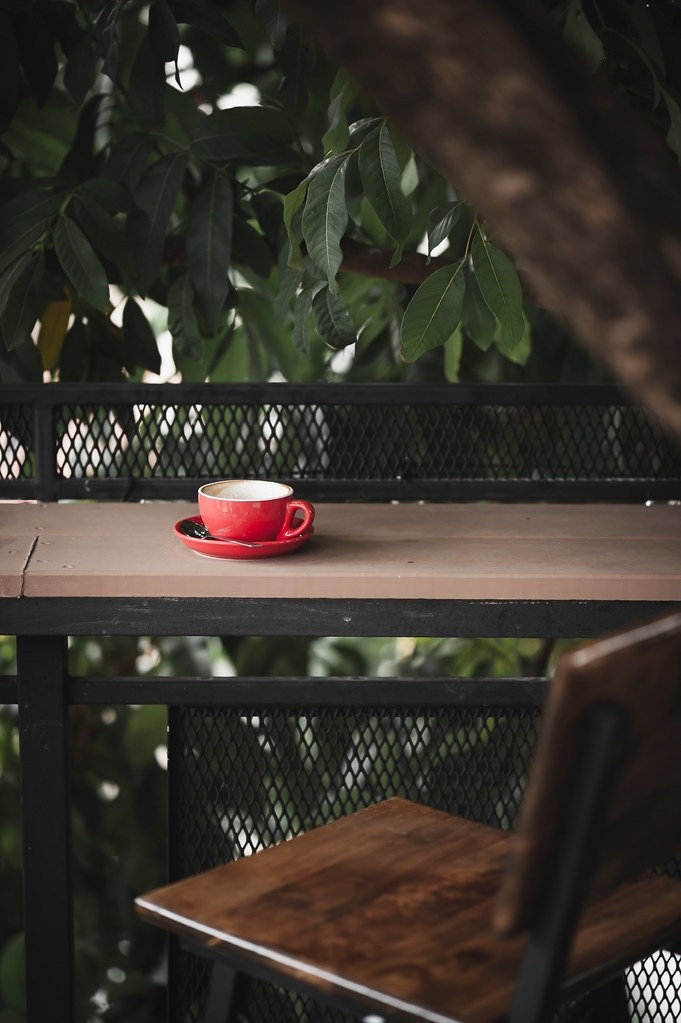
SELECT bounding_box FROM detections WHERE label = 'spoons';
[178,519,262,547]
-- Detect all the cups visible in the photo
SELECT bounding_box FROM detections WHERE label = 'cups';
[198,480,315,541]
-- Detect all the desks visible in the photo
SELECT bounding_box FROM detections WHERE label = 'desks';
[0,503,681,640]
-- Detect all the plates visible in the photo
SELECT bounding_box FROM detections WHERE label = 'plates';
[173,515,315,558]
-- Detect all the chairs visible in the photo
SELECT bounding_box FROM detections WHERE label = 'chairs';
[129,612,681,1023]
